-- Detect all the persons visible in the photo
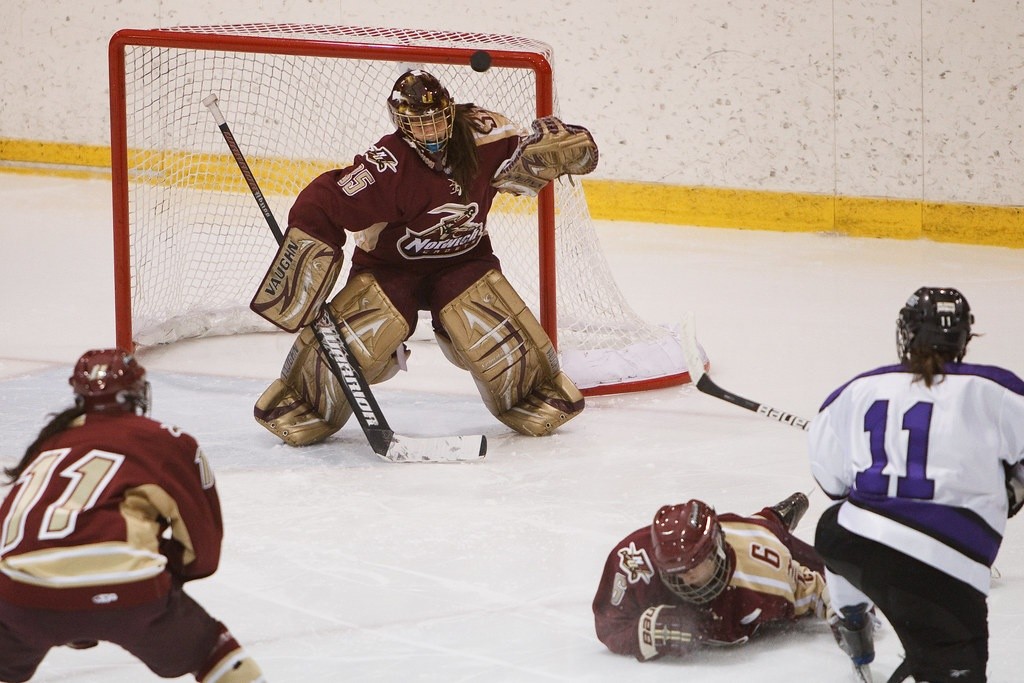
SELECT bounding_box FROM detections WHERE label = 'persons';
[251,69,599,447]
[1,349,265,683]
[808,288,1024,683]
[591,491,885,663]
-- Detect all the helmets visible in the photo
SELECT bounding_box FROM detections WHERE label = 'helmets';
[387,68,455,172]
[895,287,974,365]
[651,498,738,607]
[68,348,151,417]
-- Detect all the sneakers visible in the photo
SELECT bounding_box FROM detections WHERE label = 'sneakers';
[771,489,810,535]
[831,601,884,683]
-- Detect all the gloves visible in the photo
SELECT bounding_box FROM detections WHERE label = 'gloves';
[695,602,764,648]
[657,608,703,658]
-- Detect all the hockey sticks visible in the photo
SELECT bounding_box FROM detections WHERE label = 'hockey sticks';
[200,92,489,463]
[682,308,811,431]
